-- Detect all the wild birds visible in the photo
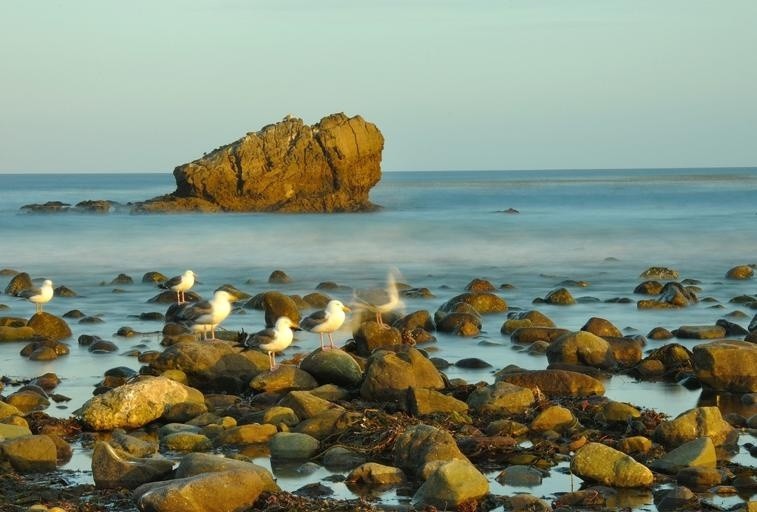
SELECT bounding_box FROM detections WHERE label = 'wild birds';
[349,264,406,332]
[156,269,198,307]
[17,278,54,316]
[299,298,352,354]
[233,316,299,374]
[171,290,241,342]
